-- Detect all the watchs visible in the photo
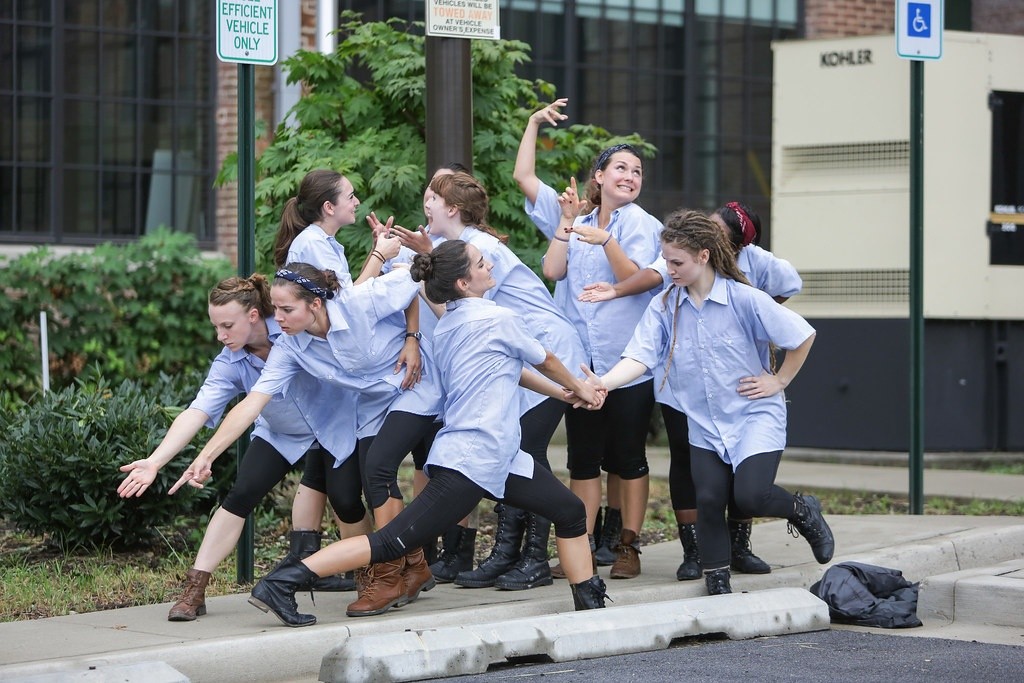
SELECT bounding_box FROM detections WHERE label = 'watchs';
[406,332,422,340]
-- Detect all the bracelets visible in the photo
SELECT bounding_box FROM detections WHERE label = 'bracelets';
[554,235,569,242]
[372,250,386,264]
[602,234,612,247]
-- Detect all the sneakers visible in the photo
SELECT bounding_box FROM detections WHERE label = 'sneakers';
[703,567,733,595]
[787,490,834,564]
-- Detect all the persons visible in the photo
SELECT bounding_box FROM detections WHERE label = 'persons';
[116,98,835,628]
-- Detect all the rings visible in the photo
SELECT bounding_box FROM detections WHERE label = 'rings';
[413,372,416,376]
[398,362,402,366]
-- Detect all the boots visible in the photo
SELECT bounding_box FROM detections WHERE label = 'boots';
[427,524,477,583]
[591,506,623,566]
[494,508,554,590]
[548,533,598,579]
[247,552,317,628]
[167,568,211,621]
[569,575,606,611]
[608,528,641,579]
[288,528,358,592]
[353,566,372,597]
[674,520,703,581]
[452,503,527,588]
[726,517,772,574]
[392,546,437,607]
[346,556,408,618]
